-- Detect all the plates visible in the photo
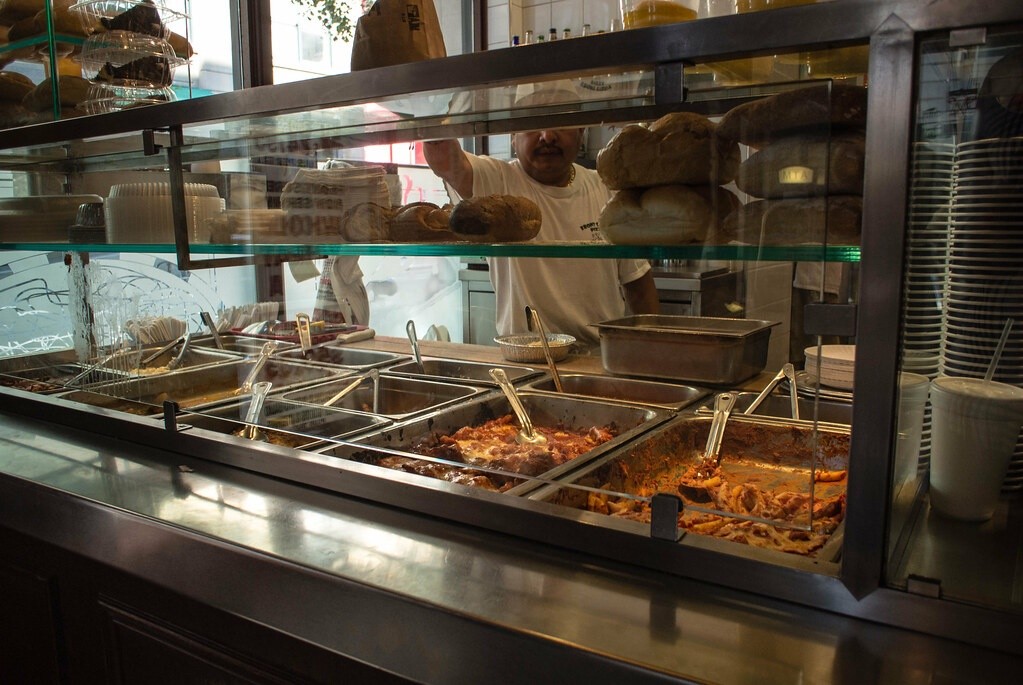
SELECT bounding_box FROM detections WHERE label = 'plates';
[925,136,1023,498]
[899,141,956,475]
[804,344,856,389]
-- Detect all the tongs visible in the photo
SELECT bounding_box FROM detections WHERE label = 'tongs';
[323,369,379,413]
[743,362,799,420]
[142,332,193,369]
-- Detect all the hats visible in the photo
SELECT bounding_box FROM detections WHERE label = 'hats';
[515,78,581,104]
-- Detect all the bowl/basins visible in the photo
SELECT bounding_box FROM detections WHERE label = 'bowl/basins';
[620,0,700,30]
[493,333,576,363]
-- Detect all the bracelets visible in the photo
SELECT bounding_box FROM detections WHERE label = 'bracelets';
[424,141,443,144]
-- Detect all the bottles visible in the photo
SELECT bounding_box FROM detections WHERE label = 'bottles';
[536,35,545,44]
[525,30,533,44]
[513,36,519,46]
[548,28,557,41]
[611,17,619,32]
[562,29,571,39]
[583,24,590,36]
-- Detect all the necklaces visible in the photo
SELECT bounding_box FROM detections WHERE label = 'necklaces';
[568,166,574,186]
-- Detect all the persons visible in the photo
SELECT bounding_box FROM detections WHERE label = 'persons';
[422,78,660,345]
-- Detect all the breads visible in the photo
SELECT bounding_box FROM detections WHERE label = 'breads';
[594,82,866,248]
[339,193,541,246]
[623,0,701,30]
[0,0,196,128]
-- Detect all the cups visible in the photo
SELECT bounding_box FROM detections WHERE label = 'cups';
[893,371,929,484]
[930,376,1023,529]
